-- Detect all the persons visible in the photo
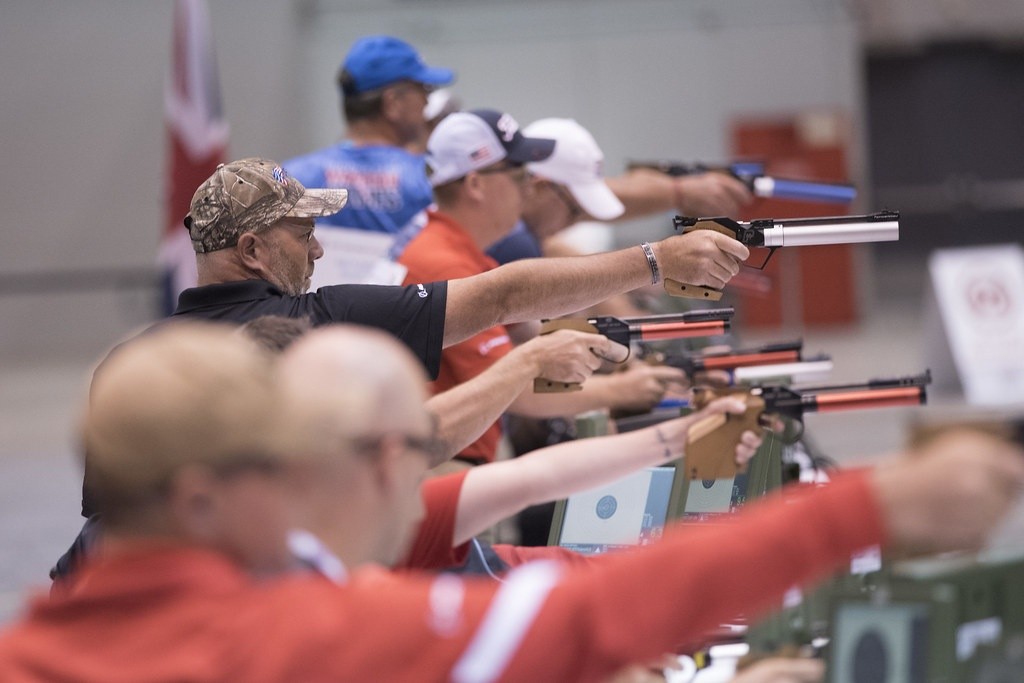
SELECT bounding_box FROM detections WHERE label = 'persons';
[50,84,783,600]
[0,319,1024,683]
[279,33,459,294]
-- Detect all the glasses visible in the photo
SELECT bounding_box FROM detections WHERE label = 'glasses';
[356,425,450,471]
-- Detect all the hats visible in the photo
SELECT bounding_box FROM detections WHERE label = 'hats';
[464,108,556,163]
[518,114,625,220]
[183,154,348,252]
[420,85,450,119]
[423,111,507,187]
[338,35,455,95]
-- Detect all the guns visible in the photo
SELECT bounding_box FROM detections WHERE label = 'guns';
[608,338,802,421]
[684,355,836,403]
[905,403,1024,502]
[660,207,904,304]
[533,305,734,394]
[684,370,934,478]
[625,156,857,203]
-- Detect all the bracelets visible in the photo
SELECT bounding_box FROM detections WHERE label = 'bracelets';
[641,241,660,284]
